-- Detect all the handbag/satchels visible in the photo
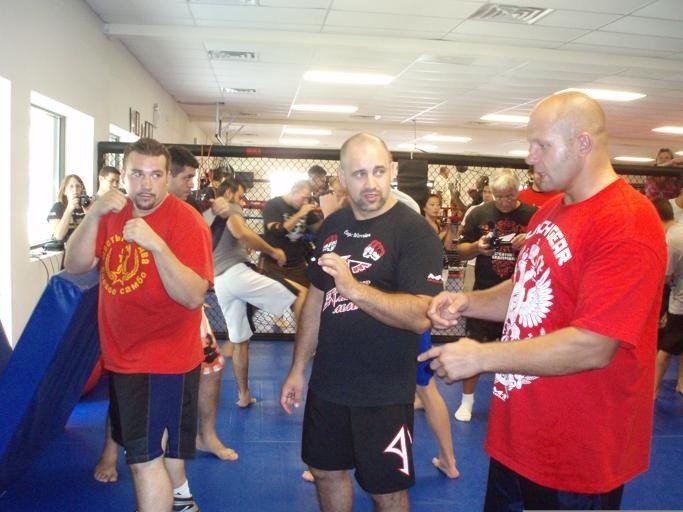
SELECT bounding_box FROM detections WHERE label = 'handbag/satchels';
[42,241,64,251]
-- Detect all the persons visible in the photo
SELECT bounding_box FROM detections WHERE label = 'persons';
[417,89,669,511]
[63,137,215,512]
[391,166,566,477]
[643,148,683,401]
[44,149,350,484]
[279,133,447,512]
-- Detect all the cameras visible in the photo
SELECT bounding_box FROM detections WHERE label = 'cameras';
[439,217,448,226]
[76,190,91,207]
[485,232,503,250]
